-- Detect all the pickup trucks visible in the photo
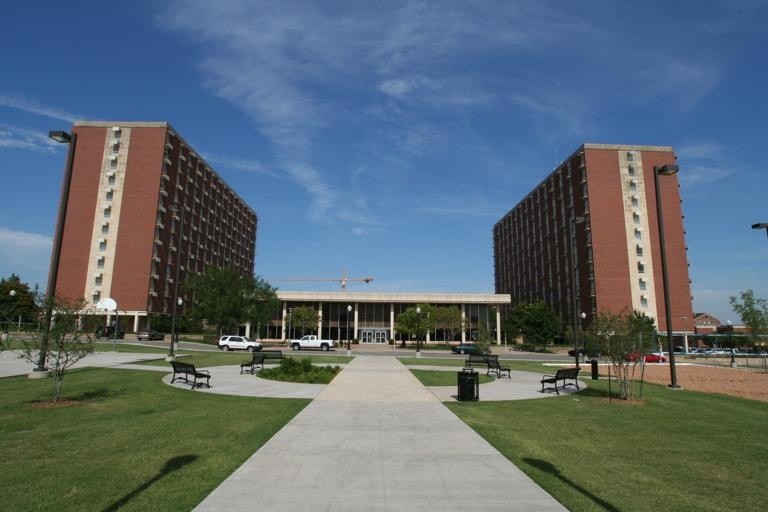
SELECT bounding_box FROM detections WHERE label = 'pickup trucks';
[290,335,333,351]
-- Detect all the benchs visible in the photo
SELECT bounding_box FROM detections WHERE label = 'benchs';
[239,350,287,375]
[464,352,512,380]
[540,366,582,396]
[169,360,211,391]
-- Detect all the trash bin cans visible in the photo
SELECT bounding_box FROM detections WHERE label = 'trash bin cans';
[457,368,479,401]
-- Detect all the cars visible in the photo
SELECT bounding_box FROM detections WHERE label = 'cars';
[452,344,491,355]
[689,347,739,354]
[568,347,600,357]
[137,328,164,341]
[626,351,666,362]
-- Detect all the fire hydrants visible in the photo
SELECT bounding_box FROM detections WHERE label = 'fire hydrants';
[591,360,598,379]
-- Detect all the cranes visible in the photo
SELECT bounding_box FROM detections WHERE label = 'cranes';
[266,270,373,291]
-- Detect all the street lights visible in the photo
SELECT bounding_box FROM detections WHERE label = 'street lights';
[653,164,683,390]
[1,290,16,340]
[166,203,184,362]
[416,307,421,358]
[581,312,586,363]
[28,131,77,379]
[569,217,585,373]
[347,305,352,352]
[288,308,292,349]
[727,320,737,367]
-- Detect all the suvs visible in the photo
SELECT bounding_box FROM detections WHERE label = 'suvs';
[217,335,263,352]
[94,326,124,340]
